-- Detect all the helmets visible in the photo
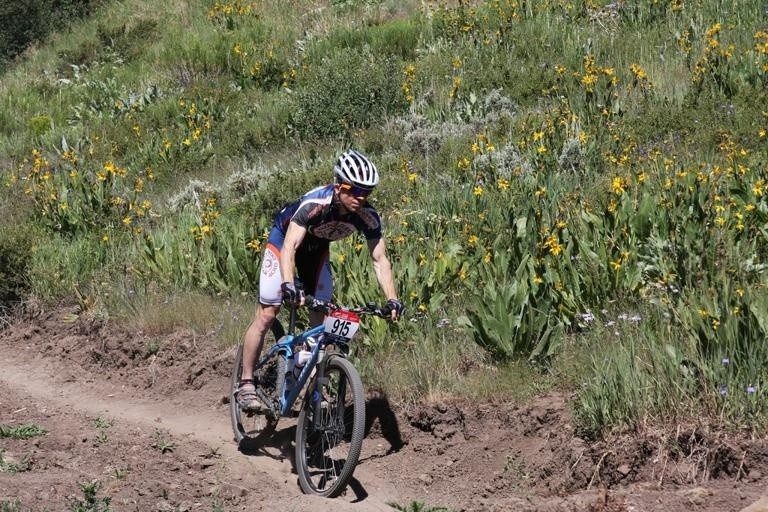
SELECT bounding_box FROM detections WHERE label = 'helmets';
[335,149,379,190]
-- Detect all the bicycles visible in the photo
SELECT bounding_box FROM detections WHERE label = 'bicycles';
[229,287,394,497]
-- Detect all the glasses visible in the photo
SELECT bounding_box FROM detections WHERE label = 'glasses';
[340,184,372,196]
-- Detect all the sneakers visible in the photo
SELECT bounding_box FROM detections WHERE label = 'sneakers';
[292,388,328,412]
[234,379,261,411]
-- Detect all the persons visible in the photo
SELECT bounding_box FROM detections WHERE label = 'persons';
[235,149,404,411]
